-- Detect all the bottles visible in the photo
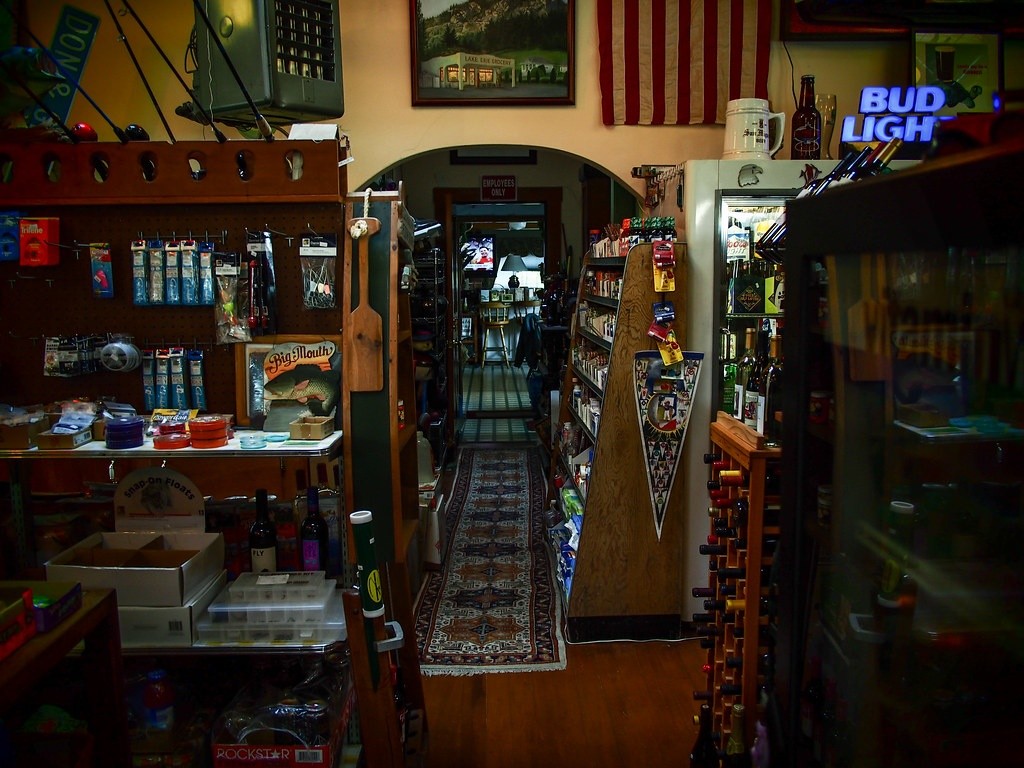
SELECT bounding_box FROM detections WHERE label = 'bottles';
[140,671,177,730]
[542,229,620,541]
[298,489,330,576]
[691,255,797,766]
[313,462,343,555]
[618,216,677,258]
[292,471,311,537]
[755,134,904,267]
[248,490,279,574]
[790,74,822,160]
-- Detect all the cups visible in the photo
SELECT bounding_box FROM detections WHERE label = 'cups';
[813,93,838,159]
[719,97,787,161]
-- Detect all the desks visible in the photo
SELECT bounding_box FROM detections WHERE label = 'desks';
[0,588,132,768]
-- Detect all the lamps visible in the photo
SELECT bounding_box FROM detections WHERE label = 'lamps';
[501,255,529,288]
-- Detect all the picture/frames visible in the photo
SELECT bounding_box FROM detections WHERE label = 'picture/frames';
[409,0,575,106]
[779,0,1024,42]
[450,149,538,164]
[908,27,1005,115]
[235,334,342,428]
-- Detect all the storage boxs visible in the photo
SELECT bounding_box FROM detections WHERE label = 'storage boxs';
[0,211,60,266]
[1,412,107,450]
[289,417,334,439]
[43,531,347,647]
[208,663,352,768]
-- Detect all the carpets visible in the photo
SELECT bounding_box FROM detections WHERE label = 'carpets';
[413,446,567,676]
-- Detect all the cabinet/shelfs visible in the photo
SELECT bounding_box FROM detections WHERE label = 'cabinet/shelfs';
[544,240,688,642]
[414,248,447,471]
[0,139,355,656]
[708,410,781,768]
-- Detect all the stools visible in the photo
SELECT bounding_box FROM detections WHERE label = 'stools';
[480,300,541,369]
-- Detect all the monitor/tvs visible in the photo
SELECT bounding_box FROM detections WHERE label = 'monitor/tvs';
[463,234,497,279]
